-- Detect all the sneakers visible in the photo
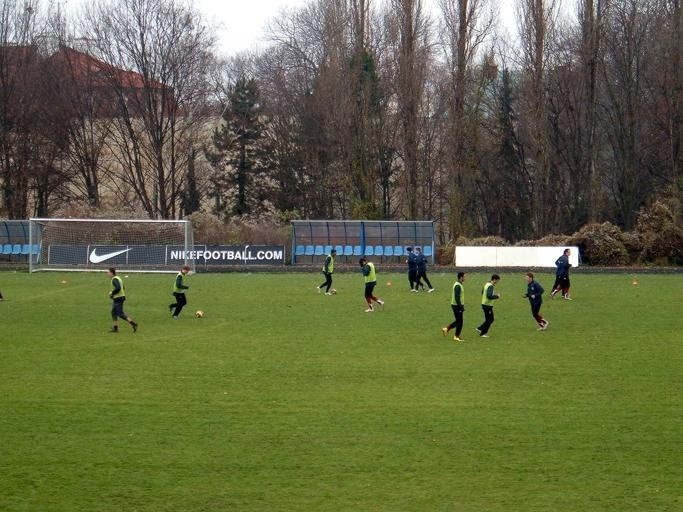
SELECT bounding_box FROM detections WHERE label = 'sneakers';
[476,327,489,337]
[317,286,332,296]
[410,284,435,293]
[109,323,138,333]
[365,301,384,313]
[442,327,464,342]
[537,322,549,332]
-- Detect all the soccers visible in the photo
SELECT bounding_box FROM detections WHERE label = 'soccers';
[331,289,337,294]
[195,311,204,319]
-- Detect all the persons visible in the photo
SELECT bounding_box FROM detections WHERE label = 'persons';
[317,250,336,296]
[107,267,138,332]
[168,266,189,319]
[477,275,501,339]
[410,247,441,293]
[441,272,469,341]
[549,249,573,300]
[406,246,426,294]
[522,273,549,331]
[359,254,384,312]
